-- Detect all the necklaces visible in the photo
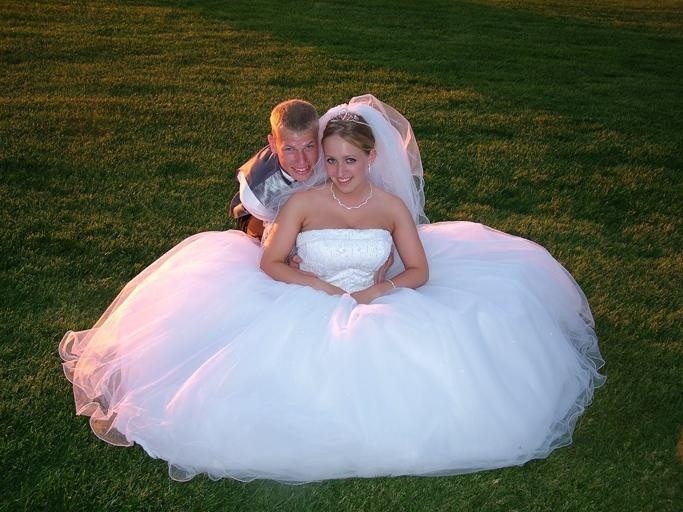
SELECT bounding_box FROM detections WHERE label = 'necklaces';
[330,181,373,210]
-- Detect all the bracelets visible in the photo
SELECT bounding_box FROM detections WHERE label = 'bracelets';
[387,279,397,290]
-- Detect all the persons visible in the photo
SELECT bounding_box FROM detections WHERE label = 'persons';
[258,112,430,306]
[228,98,395,283]
